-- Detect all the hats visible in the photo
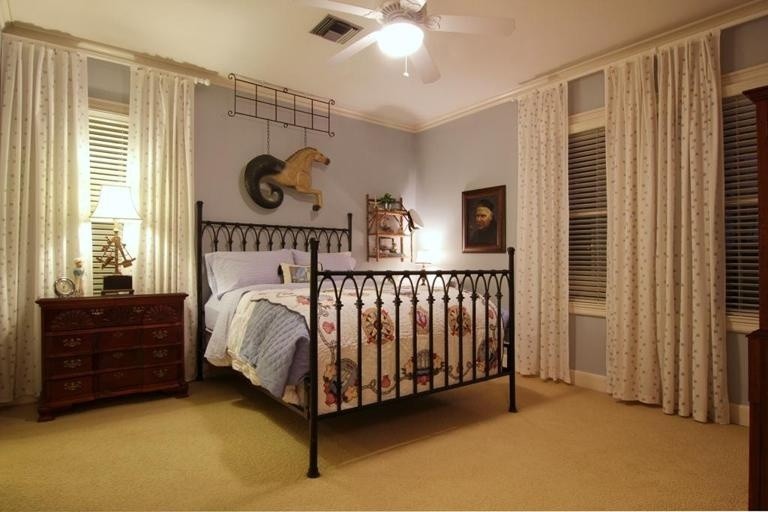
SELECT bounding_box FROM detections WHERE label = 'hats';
[476,199,495,212]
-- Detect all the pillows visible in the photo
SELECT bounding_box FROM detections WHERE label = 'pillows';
[203,249,354,300]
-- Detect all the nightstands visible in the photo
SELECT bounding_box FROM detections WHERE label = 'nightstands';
[34,292,189,422]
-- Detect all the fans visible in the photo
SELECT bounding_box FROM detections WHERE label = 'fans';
[311,0,515,85]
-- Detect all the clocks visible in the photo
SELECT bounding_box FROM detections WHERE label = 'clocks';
[53,278,75,298]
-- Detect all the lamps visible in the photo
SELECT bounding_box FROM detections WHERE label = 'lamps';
[89,183,143,295]
[414,249,432,285]
[377,18,424,78]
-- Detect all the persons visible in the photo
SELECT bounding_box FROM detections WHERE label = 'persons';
[73,257,85,298]
[466,198,498,247]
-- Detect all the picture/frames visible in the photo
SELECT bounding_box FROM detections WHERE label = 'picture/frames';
[461,183,506,254]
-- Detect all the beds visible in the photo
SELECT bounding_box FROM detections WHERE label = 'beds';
[195,199,518,477]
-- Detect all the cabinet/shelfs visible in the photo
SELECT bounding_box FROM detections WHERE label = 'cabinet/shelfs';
[740,85,767,511]
[365,194,404,262]
